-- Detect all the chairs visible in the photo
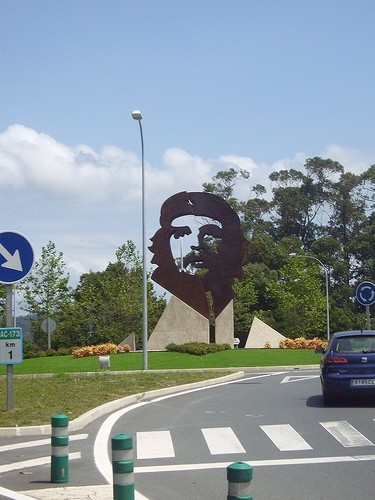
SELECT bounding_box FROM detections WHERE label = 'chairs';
[338,340,352,351]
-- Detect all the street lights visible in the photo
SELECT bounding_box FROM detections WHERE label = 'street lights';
[288,251,331,343]
[130,109,153,371]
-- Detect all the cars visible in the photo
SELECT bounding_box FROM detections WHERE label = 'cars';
[320,330,375,407]
